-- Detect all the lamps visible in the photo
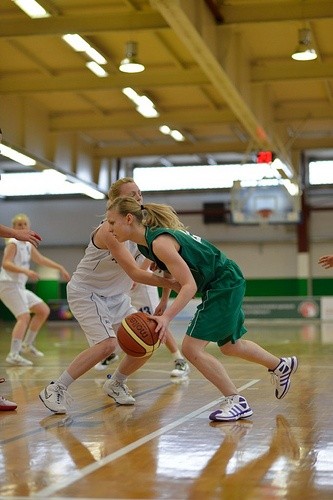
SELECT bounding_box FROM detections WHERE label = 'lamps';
[291,29,318,62]
[119,41,146,74]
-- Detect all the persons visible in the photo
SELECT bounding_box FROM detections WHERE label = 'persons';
[92,237,190,376]
[107,198,299,420]
[317,256,333,269]
[0,213,72,367]
[0,224,43,411]
[38,176,154,414]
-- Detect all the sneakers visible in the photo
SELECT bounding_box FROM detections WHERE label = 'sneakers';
[19,343,44,358]
[102,379,136,405]
[39,381,74,414]
[6,353,33,367]
[94,353,119,371]
[267,356,298,400]
[209,394,253,421]
[170,358,190,377]
[0,396,18,411]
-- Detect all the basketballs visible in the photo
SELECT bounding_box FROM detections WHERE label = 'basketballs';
[116,312,161,359]
